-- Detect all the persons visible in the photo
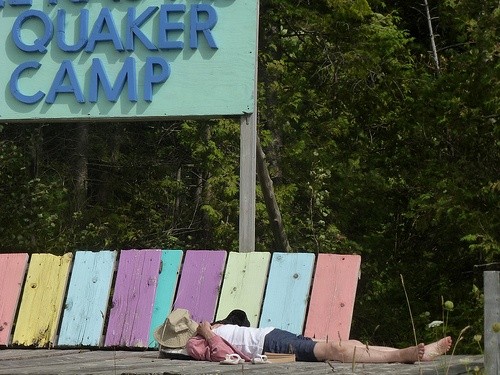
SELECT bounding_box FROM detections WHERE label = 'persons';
[153,308,453,365]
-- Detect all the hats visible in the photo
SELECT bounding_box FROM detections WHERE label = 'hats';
[153,309,200,348]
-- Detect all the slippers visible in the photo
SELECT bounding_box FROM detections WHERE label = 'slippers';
[217,353,246,364]
[251,353,271,364]
